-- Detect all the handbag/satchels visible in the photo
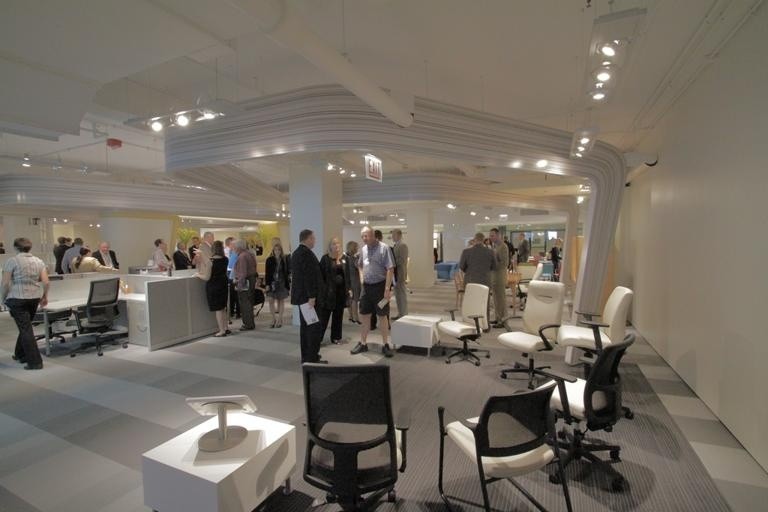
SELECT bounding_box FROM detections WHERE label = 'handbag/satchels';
[251,288,265,317]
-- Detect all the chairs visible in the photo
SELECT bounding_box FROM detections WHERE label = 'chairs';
[67,278,130,357]
[30,274,78,349]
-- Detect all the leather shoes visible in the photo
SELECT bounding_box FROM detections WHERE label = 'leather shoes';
[11,355,43,370]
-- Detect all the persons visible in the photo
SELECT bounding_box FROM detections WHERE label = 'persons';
[151,227,407,365]
[434,248,438,264]
[459,228,531,333]
[0,237,50,369]
[52,233,120,274]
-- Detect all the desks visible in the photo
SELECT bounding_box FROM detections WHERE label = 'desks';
[142,413,297,512]
[45,291,146,356]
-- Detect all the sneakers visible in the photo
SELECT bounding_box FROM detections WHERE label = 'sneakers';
[391,316,399,320]
[382,343,394,358]
[350,341,369,354]
[347,318,363,327]
[490,319,505,328]
[330,336,343,345]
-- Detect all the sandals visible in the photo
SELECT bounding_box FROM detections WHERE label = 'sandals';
[213,321,282,337]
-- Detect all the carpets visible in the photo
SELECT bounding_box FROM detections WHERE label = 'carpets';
[264,343,732,512]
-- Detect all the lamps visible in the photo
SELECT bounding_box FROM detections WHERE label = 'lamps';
[577,2,647,100]
[569,108,599,159]
[124,57,246,133]
